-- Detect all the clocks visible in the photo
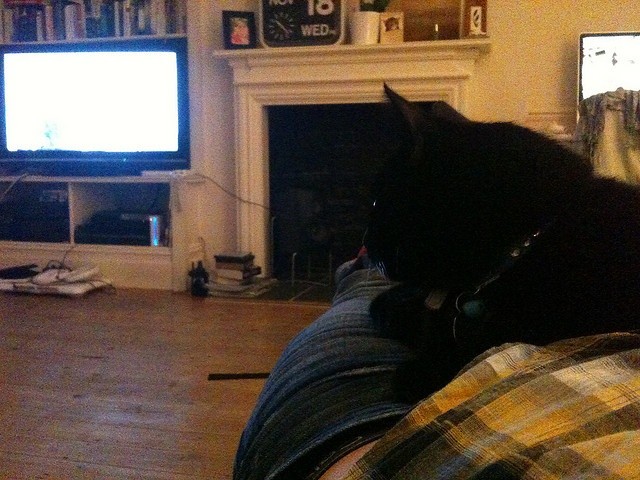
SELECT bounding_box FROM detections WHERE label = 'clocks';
[259,0,346,48]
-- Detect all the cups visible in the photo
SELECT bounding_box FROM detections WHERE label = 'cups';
[347,11,381,46]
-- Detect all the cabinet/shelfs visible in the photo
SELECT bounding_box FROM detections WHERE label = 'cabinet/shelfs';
[0,173,205,292]
[0,0,196,45]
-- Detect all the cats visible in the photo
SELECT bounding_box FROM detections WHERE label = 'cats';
[360,81,639,407]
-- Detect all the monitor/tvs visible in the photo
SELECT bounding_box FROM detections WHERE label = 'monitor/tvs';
[578,32,640,124]
[0,40,189,175]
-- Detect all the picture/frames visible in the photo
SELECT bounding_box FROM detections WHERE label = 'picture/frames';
[222,9,256,49]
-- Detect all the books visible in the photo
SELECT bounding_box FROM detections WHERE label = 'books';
[1,1,180,42]
[205,252,275,298]
